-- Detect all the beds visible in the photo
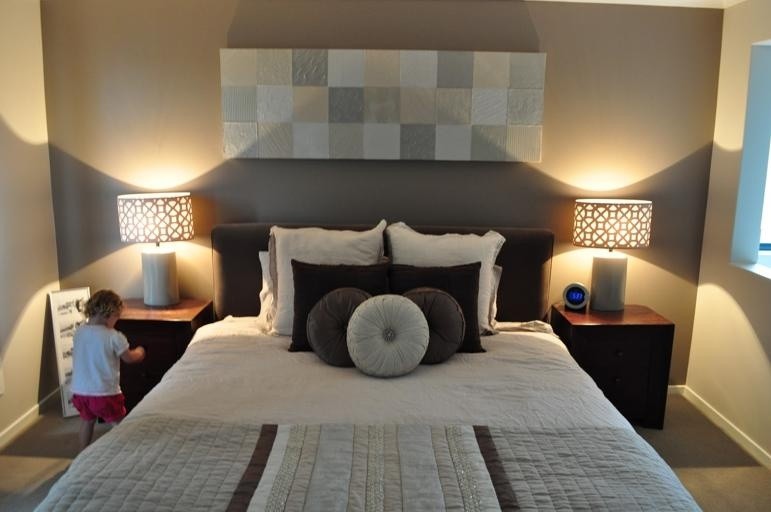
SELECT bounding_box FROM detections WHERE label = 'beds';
[32,224,706,512]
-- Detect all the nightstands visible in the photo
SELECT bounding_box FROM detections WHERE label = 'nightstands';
[114,298,214,414]
[551,305,674,429]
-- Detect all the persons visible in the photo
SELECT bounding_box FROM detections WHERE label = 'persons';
[69,288,145,451]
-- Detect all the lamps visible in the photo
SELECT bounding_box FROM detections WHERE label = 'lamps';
[116,192,195,307]
[572,198,652,311]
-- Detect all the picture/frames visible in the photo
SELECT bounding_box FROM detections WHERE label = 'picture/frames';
[48,287,91,417]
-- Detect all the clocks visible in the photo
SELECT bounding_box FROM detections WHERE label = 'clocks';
[563,283,589,314]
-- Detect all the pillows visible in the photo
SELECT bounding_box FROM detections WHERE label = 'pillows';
[489,265,501,330]
[256,251,273,330]
[385,224,504,335]
[402,288,465,365]
[268,219,385,336]
[348,295,429,378]
[289,260,390,353]
[306,287,372,367]
[386,262,486,352]
